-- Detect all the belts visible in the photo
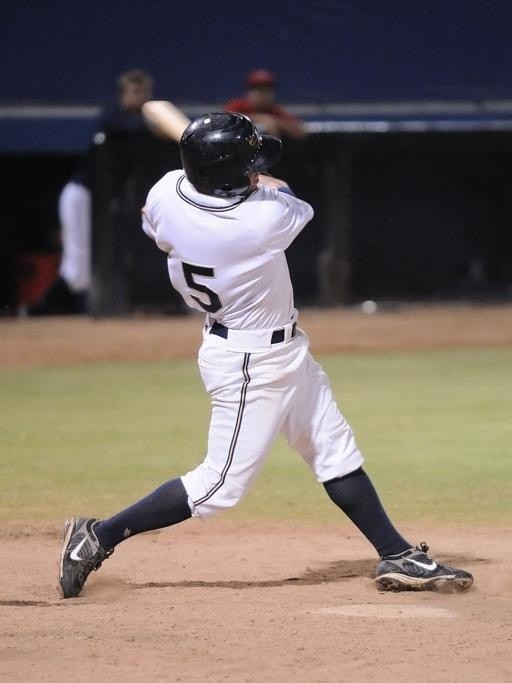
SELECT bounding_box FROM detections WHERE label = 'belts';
[209,319,297,345]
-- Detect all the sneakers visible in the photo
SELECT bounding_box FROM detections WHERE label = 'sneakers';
[58,516,114,599]
[374,542,473,593]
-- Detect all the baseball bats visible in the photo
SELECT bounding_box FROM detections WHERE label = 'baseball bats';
[141,102,189,144]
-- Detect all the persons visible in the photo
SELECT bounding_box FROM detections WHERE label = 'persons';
[101,66,156,129]
[58,112,475,599]
[222,70,308,145]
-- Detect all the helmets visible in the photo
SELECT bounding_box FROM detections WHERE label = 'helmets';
[179,111,283,197]
